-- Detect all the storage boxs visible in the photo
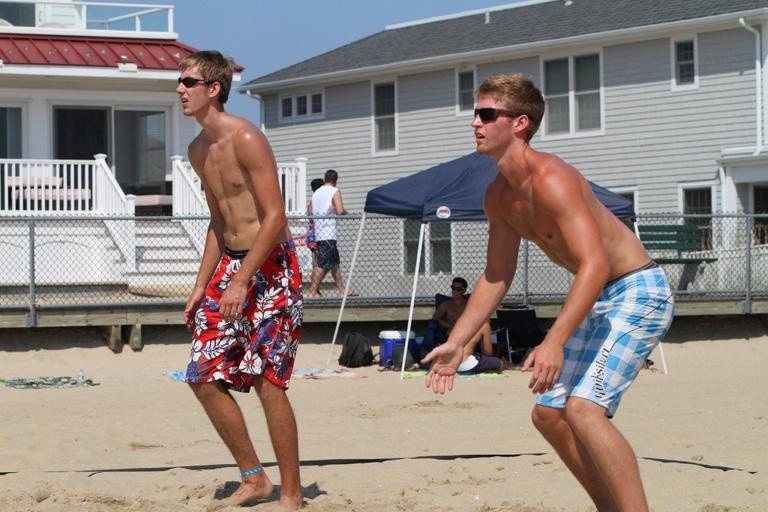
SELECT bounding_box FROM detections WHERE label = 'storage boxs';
[378,330,416,367]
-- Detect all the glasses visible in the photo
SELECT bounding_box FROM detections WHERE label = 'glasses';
[178,77,205,88]
[474,108,522,122]
[451,285,465,292]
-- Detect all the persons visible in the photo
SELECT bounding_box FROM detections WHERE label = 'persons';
[420,71,673,512]
[420,276,504,377]
[305,170,359,299]
[176,50,304,512]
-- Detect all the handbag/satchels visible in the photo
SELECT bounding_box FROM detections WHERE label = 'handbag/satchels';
[338,332,373,368]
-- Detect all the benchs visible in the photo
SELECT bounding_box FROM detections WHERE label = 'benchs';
[638,224,719,289]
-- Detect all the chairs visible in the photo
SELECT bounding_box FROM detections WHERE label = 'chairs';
[490,308,542,365]
[435,294,470,319]
